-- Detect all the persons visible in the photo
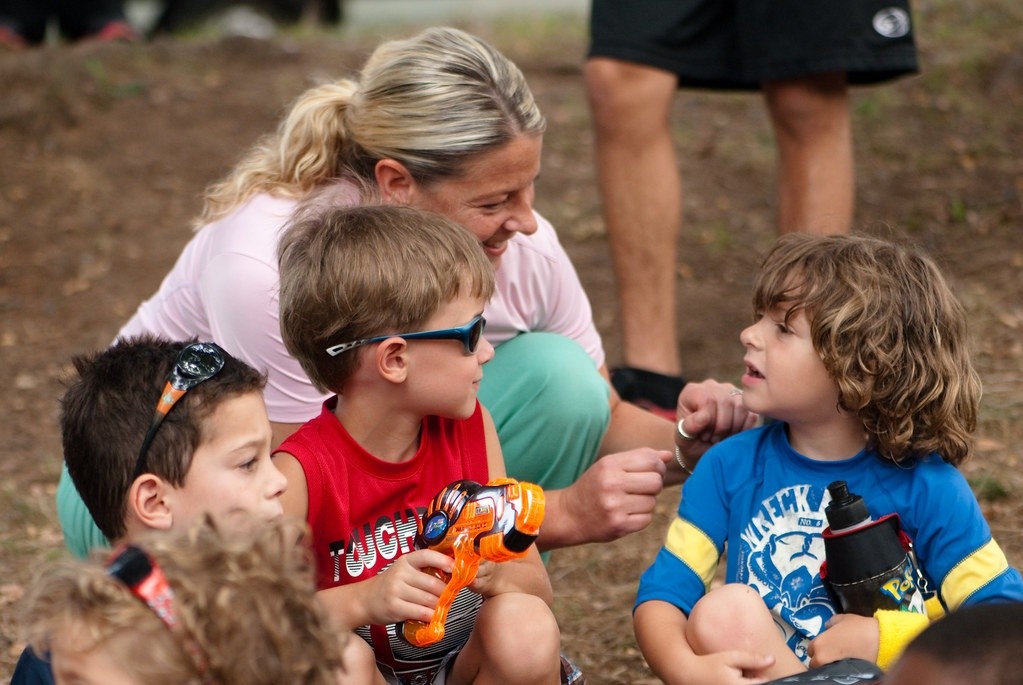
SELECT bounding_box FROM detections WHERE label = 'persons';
[580,0,922,423]
[12,334,383,685]
[637,230,1023,685]
[58,29,762,569]
[267,209,588,684]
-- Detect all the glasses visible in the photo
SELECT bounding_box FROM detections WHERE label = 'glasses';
[326,315,486,357]
[132,342,231,486]
[103,542,219,685]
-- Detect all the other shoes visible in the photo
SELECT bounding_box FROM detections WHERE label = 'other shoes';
[606,367,685,424]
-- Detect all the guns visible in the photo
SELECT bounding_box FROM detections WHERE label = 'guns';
[396,478,544,648]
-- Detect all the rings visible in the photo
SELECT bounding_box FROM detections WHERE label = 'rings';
[676,418,698,440]
[728,389,742,395]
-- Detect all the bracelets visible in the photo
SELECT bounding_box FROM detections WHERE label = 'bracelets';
[870,608,931,671]
[675,447,694,474]
